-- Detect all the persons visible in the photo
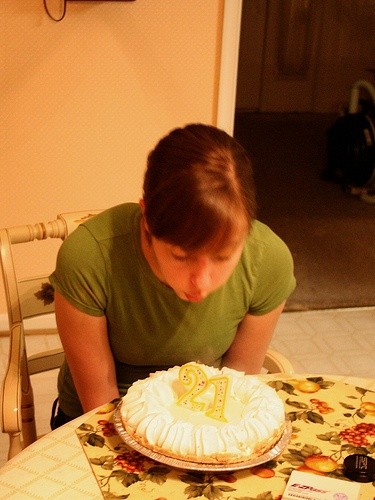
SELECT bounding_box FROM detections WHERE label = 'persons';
[48,124,297,430]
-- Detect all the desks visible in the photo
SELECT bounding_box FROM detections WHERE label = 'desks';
[0,372,375,500]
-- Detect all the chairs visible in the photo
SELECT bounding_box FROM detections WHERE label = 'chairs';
[0,209,295,461]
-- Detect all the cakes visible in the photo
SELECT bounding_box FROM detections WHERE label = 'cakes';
[120,362,287,465]
